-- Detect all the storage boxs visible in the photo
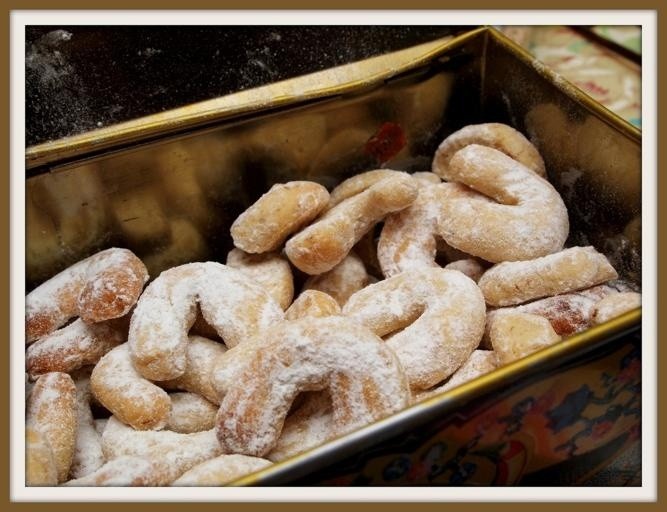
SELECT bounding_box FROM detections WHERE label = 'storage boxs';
[25,26,642,486]
